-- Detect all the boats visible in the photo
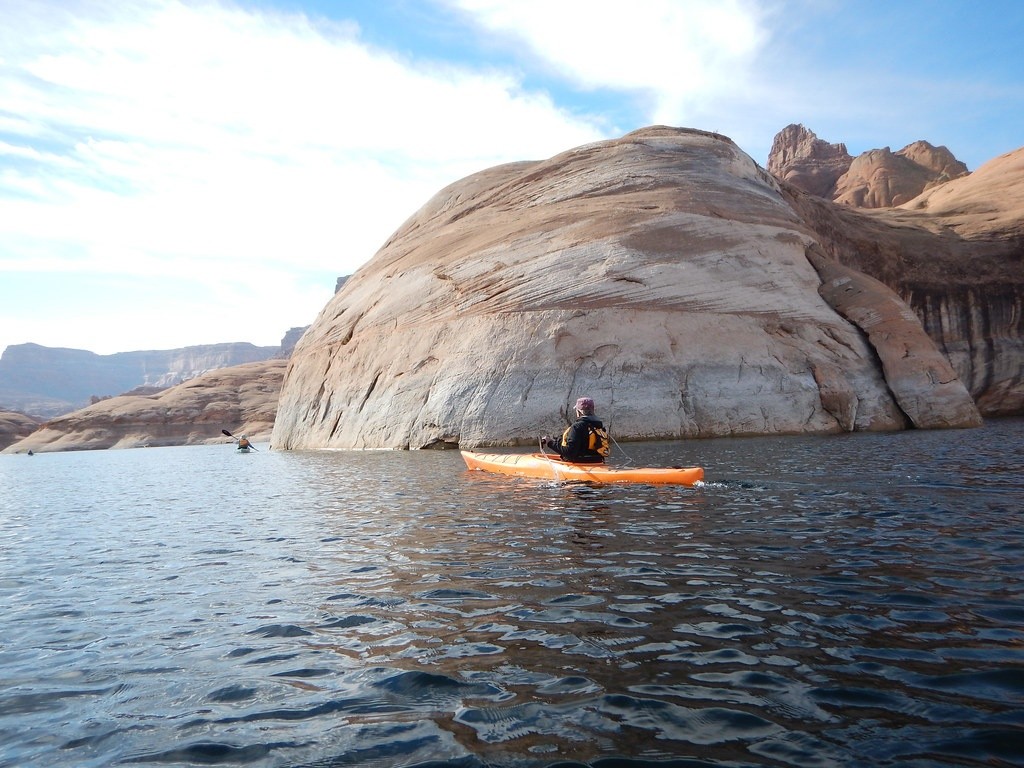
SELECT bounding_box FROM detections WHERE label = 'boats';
[232,447,255,453]
[459,450,703,484]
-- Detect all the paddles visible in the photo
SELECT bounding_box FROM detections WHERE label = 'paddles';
[221,429,259,452]
[542,435,558,444]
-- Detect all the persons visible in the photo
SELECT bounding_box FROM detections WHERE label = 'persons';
[236,434,251,449]
[541,397,610,463]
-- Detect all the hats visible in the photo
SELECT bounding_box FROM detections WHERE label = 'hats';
[240,434,247,439]
[573,397,594,412]
